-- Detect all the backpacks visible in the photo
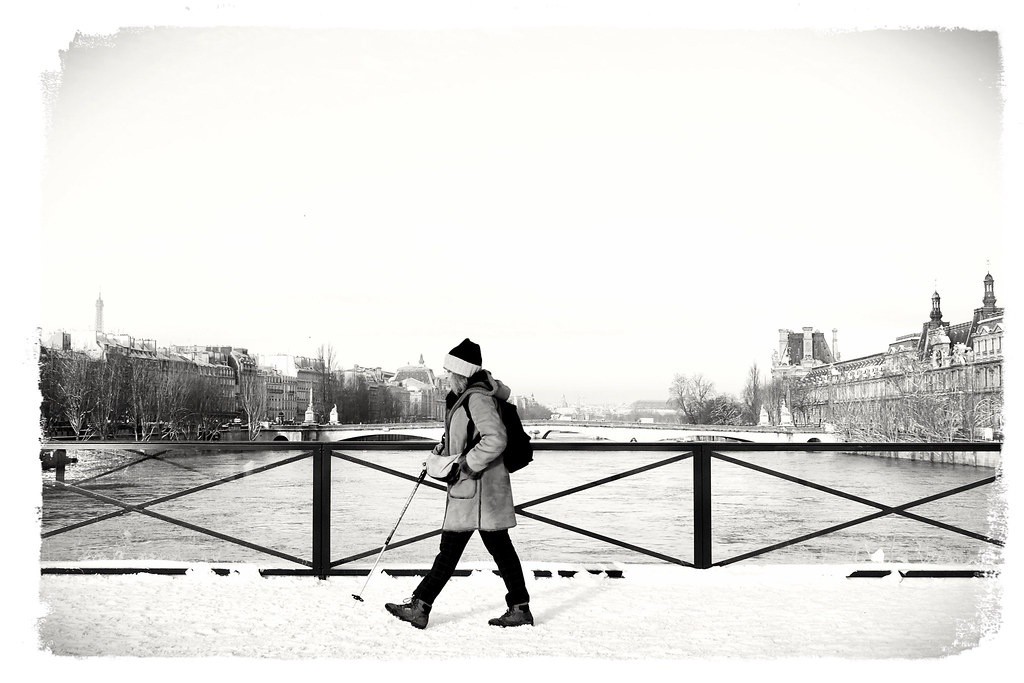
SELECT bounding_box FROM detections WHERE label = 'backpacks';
[464,394,533,473]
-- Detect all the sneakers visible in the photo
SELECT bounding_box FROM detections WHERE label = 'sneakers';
[488,602,534,627]
[385,596,432,630]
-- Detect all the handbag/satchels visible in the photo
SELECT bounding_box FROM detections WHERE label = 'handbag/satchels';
[427,450,466,482]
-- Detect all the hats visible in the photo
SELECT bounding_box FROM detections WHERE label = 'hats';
[443,338,482,377]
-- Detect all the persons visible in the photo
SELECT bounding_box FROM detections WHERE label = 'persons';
[385,338,533,627]
